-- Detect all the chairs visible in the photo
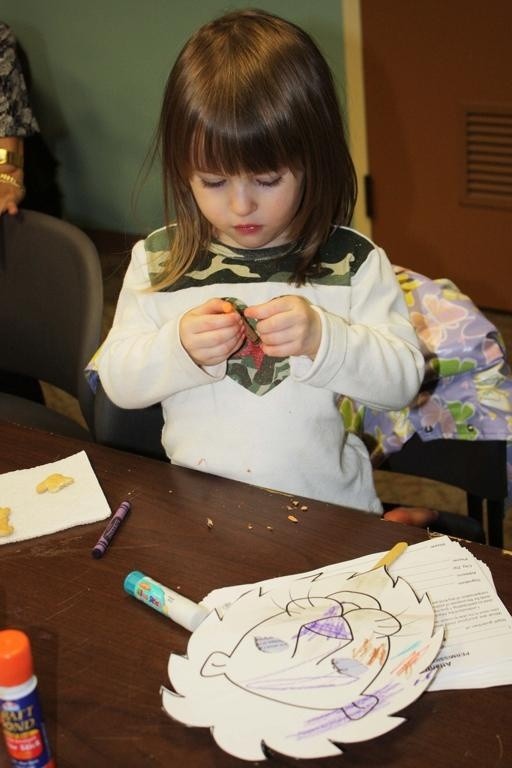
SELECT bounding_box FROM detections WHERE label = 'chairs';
[0,211,101,427]
[91,362,165,456]
[362,262,510,544]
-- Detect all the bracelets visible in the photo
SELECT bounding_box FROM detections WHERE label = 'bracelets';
[1,172,28,195]
[1,148,33,168]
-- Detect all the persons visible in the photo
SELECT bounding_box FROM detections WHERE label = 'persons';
[1,22,41,220]
[86,4,425,517]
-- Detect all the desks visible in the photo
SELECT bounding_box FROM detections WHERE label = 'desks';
[0,392,510,762]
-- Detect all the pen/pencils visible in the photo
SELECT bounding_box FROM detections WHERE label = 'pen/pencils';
[372,540,407,575]
[221,300,262,345]
[92,499,133,558]
[122,572,210,633]
